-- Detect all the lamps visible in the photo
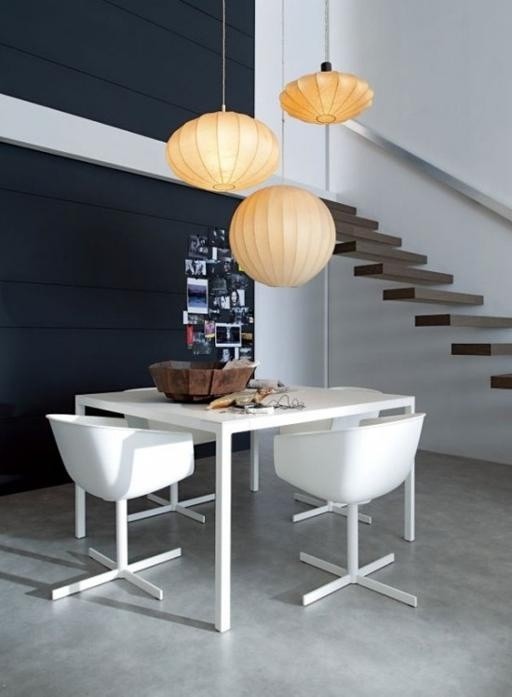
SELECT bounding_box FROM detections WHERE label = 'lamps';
[278,0,376,124]
[164,0,282,193]
[229,1,337,289]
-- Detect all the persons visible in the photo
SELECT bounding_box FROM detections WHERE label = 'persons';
[222,348,234,363]
[229,291,242,307]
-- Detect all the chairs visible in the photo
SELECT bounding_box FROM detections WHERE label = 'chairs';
[272,411,428,610]
[290,385,383,525]
[123,386,216,525]
[44,411,197,603]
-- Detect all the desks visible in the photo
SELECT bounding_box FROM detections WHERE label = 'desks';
[73,388,417,637]
